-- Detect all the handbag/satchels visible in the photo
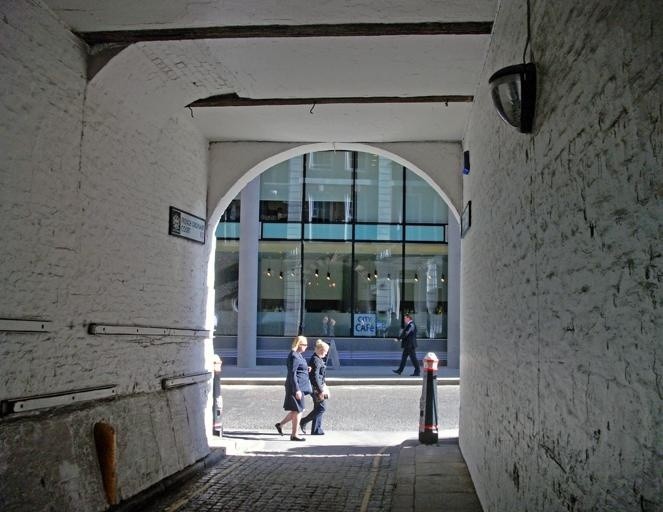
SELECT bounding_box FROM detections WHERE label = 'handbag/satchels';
[313,383,330,402]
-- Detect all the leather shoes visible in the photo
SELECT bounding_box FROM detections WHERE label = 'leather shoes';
[275,423,283,436]
[393,370,401,375]
[410,374,420,376]
[291,419,324,440]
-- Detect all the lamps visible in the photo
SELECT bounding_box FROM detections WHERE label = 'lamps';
[488,0,537,134]
[266,255,445,282]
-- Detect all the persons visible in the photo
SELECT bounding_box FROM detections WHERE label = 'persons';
[275,335,312,441]
[300,338,330,435]
[392,313,421,376]
[319,314,336,337]
